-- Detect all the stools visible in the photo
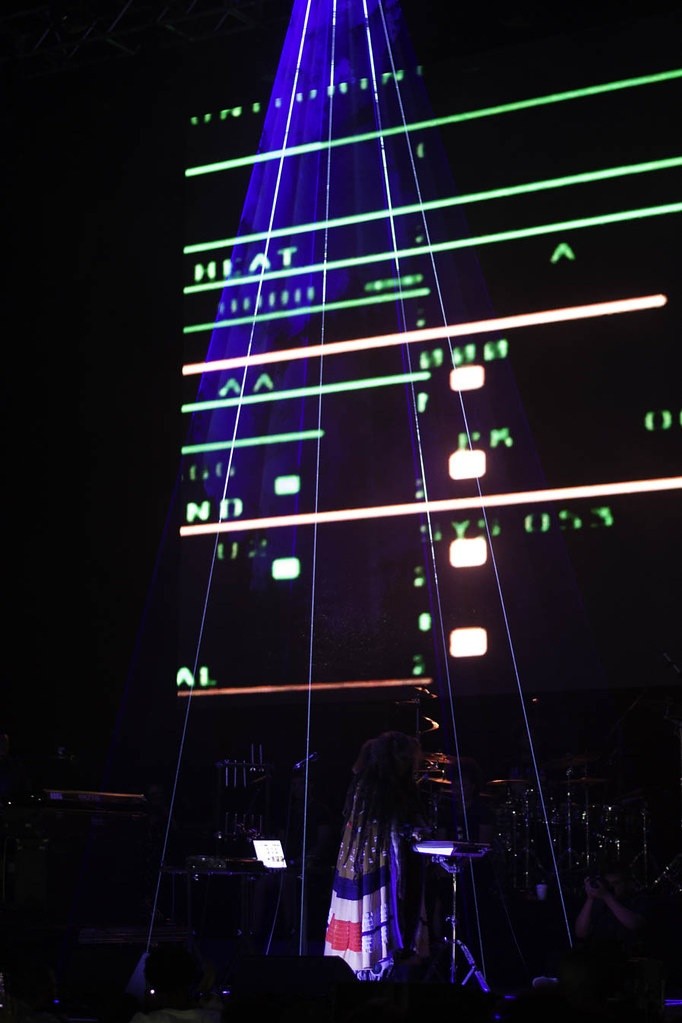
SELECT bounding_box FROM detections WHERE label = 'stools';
[408,839,494,990]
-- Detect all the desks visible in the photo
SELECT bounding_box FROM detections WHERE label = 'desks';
[169,864,308,948]
